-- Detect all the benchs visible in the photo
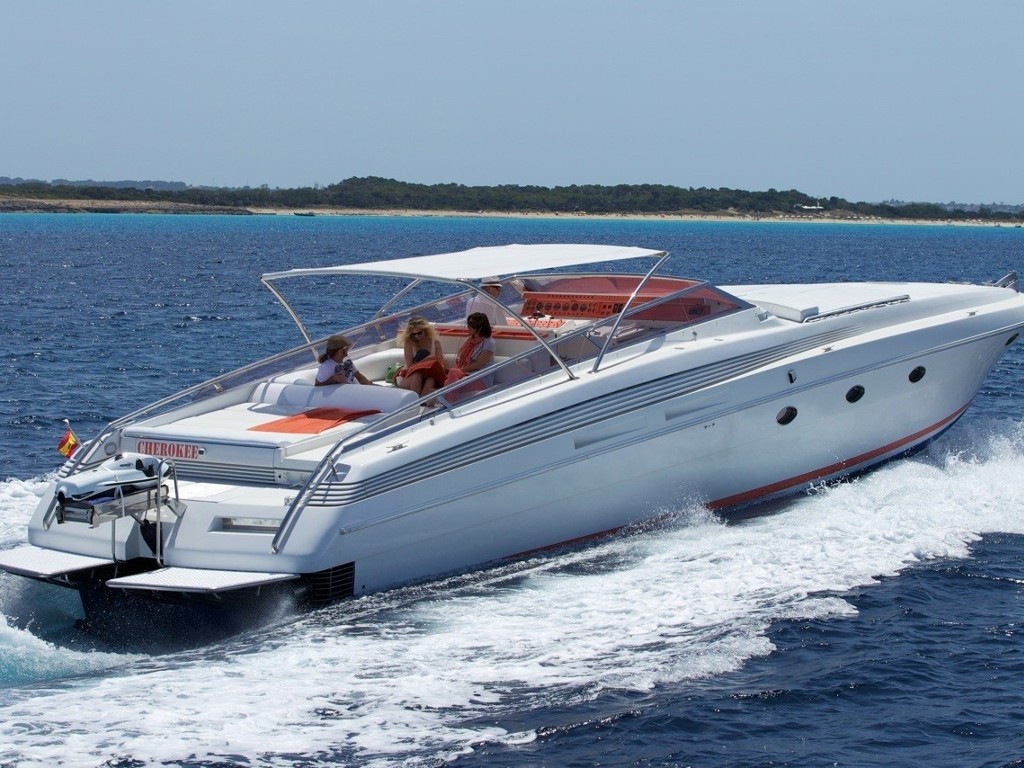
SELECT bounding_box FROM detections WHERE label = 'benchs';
[248,323,555,415]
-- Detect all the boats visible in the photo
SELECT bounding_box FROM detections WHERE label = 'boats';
[0,243,1024,636]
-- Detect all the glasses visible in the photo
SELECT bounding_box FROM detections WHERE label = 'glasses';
[412,331,422,335]
[490,286,501,291]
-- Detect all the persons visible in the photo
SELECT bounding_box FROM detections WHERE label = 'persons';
[315,335,379,386]
[465,275,508,336]
[443,312,496,404]
[398,315,443,404]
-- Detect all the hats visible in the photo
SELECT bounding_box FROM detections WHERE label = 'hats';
[327,335,351,349]
[479,274,502,287]
